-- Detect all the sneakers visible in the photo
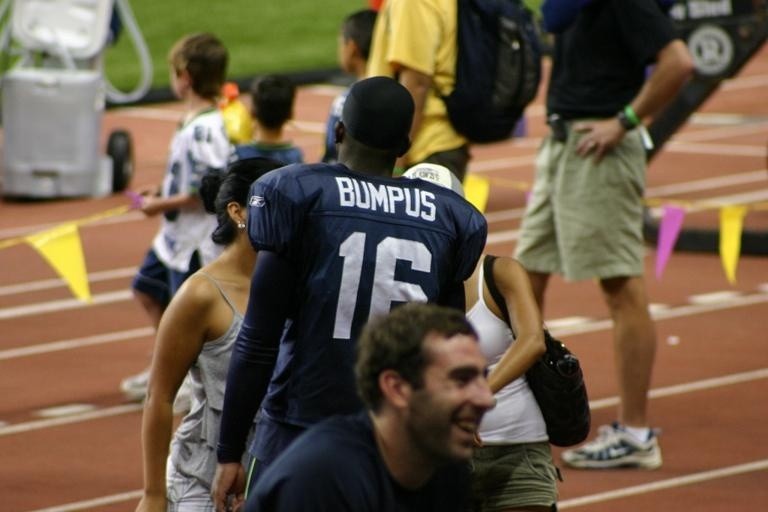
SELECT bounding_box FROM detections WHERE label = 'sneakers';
[561,421,663,470]
[122,365,194,413]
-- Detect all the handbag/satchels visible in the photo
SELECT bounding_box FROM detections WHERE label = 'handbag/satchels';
[524,329,591,448]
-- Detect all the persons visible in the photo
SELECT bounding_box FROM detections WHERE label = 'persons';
[218,75,260,147]
[121,33,230,398]
[320,12,377,166]
[240,305,496,512]
[230,73,304,164]
[515,1,699,470]
[363,0,470,191]
[400,165,557,510]
[212,77,486,511]
[133,156,289,512]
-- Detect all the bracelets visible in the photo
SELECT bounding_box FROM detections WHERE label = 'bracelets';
[616,111,634,131]
[624,105,641,125]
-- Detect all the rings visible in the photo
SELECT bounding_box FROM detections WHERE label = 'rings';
[589,141,597,149]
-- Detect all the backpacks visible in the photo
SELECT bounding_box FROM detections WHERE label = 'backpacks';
[441,0,541,144]
